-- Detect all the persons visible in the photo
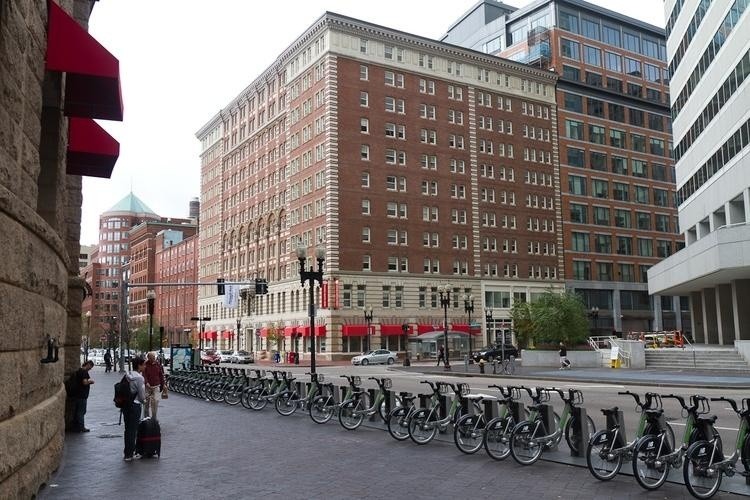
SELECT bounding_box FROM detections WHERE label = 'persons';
[72,361,95,432]
[119,357,148,460]
[142,352,166,420]
[437,344,445,366]
[557,341,572,368]
[104,349,113,373]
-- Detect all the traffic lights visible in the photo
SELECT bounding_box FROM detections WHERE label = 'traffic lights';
[263,280,268,294]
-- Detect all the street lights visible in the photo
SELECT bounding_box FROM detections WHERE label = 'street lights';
[486,307,494,343]
[295,246,325,373]
[438,283,452,370]
[591,306,600,335]
[146,290,157,351]
[237,317,241,351]
[363,305,373,351]
[84,310,92,362]
[463,294,475,364]
[191,316,211,349]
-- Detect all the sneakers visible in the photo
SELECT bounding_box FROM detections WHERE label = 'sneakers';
[123,451,143,461]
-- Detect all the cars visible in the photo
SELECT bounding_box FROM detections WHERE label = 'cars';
[350,349,399,367]
[644,334,677,349]
[91,346,257,365]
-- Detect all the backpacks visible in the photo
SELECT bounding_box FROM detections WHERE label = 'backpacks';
[65,367,82,399]
[113,374,138,425]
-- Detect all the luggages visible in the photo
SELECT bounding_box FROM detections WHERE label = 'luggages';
[135,395,162,458]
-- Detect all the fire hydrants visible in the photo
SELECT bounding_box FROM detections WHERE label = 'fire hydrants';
[478,362,484,373]
[416,353,420,360]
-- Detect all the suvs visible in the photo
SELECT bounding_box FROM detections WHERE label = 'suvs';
[470,341,518,362]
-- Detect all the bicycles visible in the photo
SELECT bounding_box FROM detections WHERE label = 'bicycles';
[509,388,597,467]
[454,384,522,454]
[484,387,564,460]
[387,381,454,440]
[407,382,473,444]
[492,355,516,376]
[164,365,401,430]
[586,388,674,482]
[632,394,721,491]
[682,396,750,498]
